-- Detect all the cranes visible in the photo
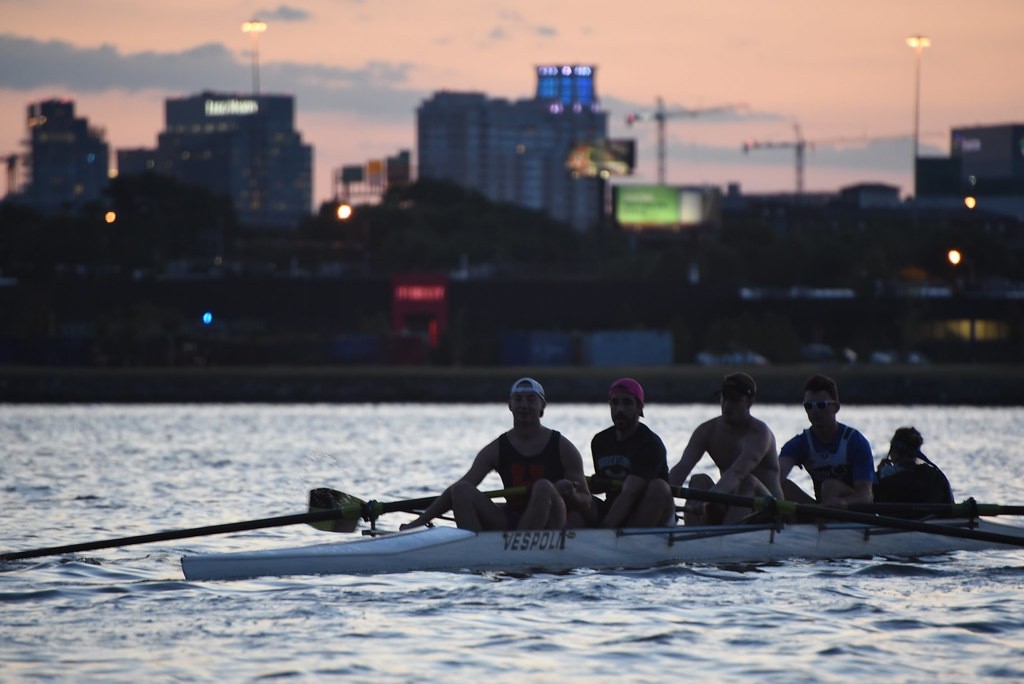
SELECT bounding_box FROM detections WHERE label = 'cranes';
[743,126,867,194]
[625,96,747,187]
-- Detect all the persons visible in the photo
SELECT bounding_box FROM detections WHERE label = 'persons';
[872,426,954,520]
[399,377,592,532]
[778,376,879,524]
[584,379,676,530]
[668,373,784,527]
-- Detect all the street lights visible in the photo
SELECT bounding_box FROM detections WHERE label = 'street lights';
[906,35,930,157]
[243,19,268,92]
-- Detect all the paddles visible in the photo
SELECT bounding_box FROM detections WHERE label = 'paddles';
[595,474,1024,549]
[825,496,1023,520]
[1,487,507,566]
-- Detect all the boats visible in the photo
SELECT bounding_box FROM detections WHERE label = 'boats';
[180,514,1023,581]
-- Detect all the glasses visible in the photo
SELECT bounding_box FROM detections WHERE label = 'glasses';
[803,400,838,410]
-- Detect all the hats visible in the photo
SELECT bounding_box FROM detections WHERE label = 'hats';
[609,379,644,418]
[511,377,546,418]
[716,371,757,399]
